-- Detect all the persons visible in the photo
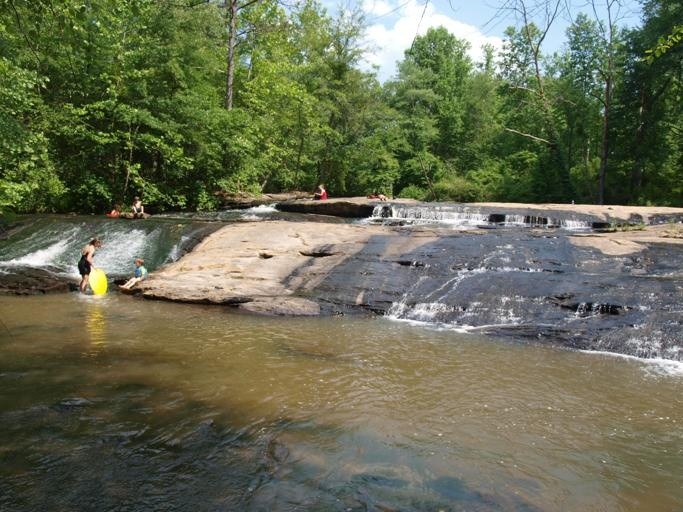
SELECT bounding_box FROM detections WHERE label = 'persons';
[132,195,145,214]
[78,239,102,292]
[315,184,328,199]
[105,204,120,218]
[118,259,148,290]
[368,193,388,201]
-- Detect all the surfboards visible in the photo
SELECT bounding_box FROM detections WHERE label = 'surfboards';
[88,268,107,294]
